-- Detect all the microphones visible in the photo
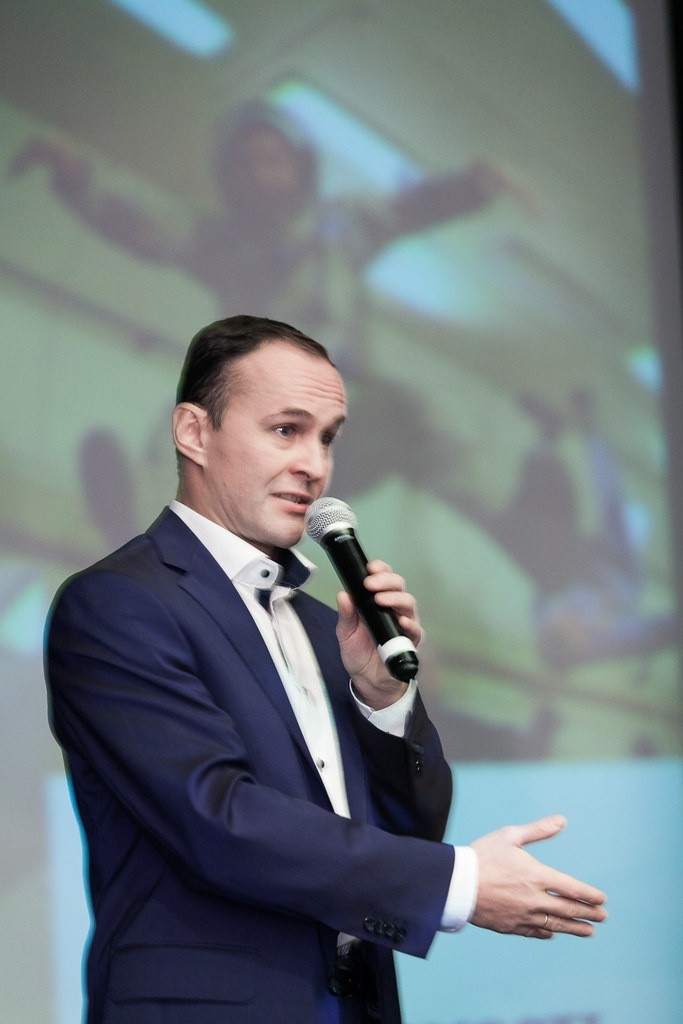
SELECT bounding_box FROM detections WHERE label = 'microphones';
[303,497,419,681]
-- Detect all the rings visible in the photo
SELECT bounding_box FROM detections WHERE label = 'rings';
[543,914,548,929]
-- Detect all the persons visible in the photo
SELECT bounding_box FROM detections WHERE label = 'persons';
[19,100,583,590]
[498,374,683,761]
[45,317,606,1024]
